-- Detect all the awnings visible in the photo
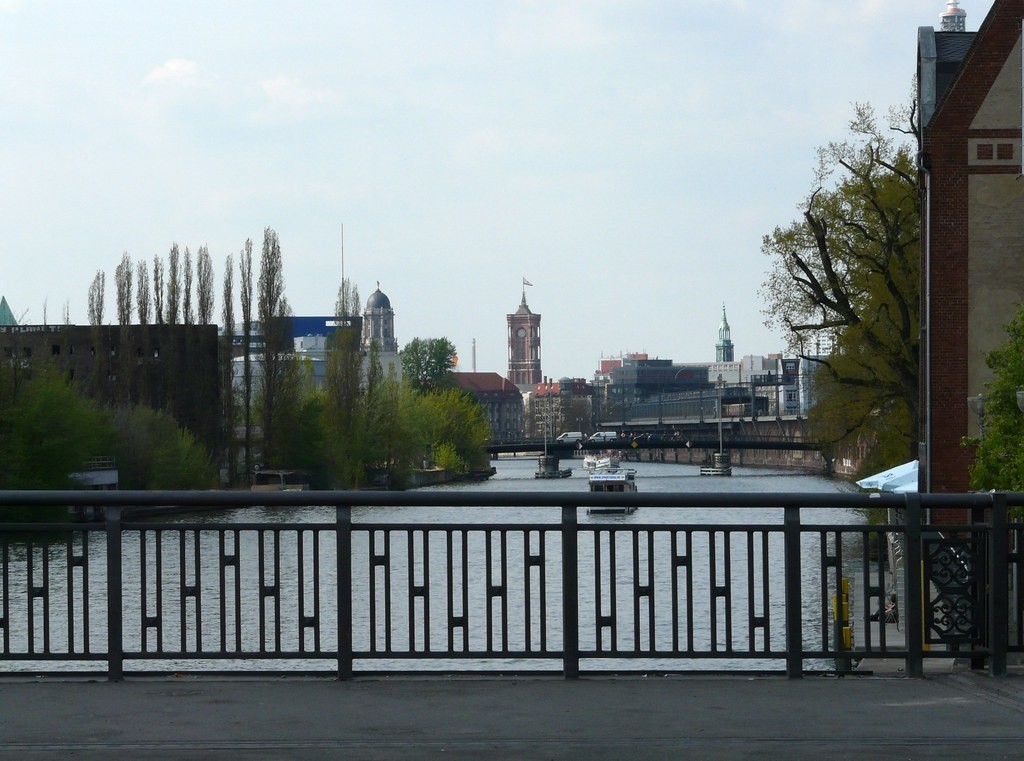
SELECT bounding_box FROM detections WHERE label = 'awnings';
[855,458,919,493]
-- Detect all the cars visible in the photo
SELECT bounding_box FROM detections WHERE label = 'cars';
[632,433,669,441]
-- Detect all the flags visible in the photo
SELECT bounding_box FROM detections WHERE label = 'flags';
[524,277,533,286]
[453,354,458,366]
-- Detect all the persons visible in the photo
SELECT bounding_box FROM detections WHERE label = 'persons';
[563,429,690,441]
[862,592,899,624]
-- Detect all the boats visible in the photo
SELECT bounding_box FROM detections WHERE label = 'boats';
[581,453,639,516]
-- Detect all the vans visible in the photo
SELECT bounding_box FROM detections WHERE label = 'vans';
[557,432,583,443]
[588,432,618,442]
